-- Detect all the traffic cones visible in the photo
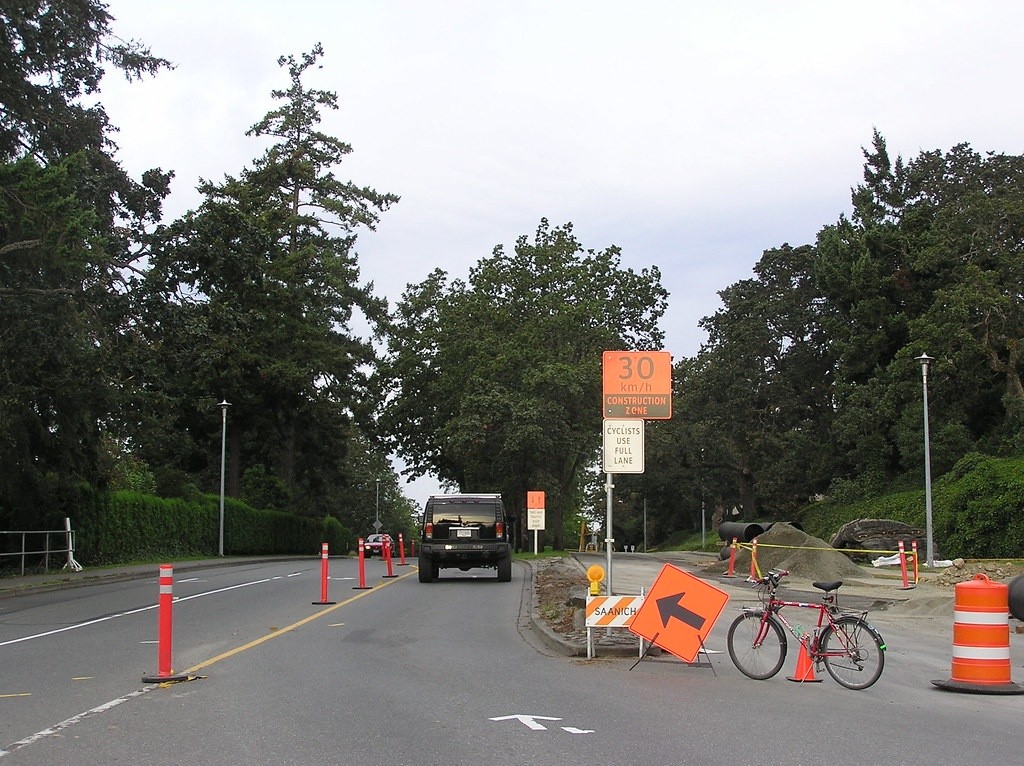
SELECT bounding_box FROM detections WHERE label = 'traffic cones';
[784,635,825,682]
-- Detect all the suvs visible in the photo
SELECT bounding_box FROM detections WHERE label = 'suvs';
[417,494,512,583]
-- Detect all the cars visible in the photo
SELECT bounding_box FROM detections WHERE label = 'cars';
[363,534,395,559]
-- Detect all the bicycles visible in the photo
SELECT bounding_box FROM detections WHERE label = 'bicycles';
[725,567,887,691]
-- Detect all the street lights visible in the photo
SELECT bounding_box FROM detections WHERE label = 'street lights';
[217,400,233,557]
[375,479,381,533]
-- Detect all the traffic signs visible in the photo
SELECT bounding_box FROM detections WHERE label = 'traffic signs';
[627,563,730,663]
